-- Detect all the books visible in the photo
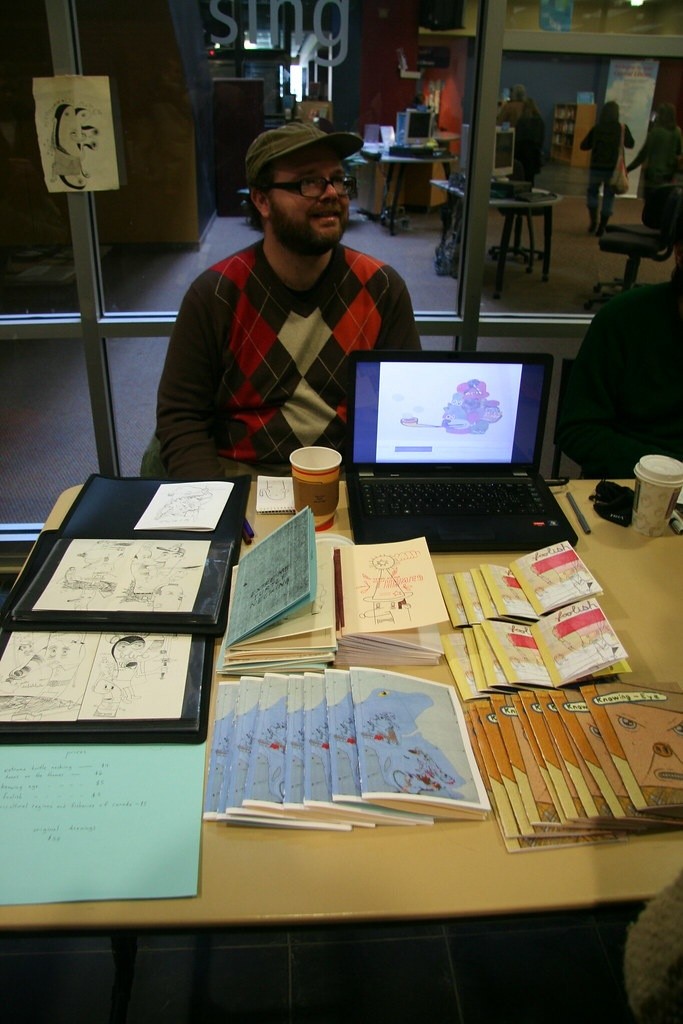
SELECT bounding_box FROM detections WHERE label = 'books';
[204,507,683,852]
[551,105,576,161]
[255,475,294,517]
[133,482,234,531]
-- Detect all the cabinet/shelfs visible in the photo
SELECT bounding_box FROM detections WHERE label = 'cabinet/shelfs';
[550,102,598,167]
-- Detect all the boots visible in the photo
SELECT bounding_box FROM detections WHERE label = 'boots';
[596,215,609,237]
[589,206,597,233]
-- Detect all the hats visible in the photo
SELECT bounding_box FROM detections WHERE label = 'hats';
[245,122,363,189]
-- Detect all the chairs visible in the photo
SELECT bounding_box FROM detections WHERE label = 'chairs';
[582,180,682,311]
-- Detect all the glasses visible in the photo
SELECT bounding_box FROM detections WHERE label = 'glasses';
[264,177,357,197]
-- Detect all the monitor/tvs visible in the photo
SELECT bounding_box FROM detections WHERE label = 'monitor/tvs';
[405,109,432,147]
[491,126,515,184]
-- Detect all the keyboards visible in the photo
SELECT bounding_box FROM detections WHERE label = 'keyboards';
[514,191,556,203]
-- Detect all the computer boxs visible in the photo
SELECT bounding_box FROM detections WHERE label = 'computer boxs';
[490,180,534,199]
[389,145,434,159]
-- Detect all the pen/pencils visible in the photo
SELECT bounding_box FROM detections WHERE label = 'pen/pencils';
[566,492,592,533]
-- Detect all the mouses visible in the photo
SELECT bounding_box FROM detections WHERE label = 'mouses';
[548,192,558,198]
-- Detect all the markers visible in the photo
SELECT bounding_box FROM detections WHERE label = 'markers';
[670,514,683,535]
[242,519,254,545]
[545,477,570,486]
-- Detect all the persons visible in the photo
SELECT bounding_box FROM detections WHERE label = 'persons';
[501,85,526,124]
[510,101,550,185]
[581,101,635,236]
[626,103,683,197]
[153,121,421,478]
[552,194,683,478]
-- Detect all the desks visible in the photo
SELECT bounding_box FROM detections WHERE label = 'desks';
[429,178,560,299]
[0,478,683,1024]
[376,153,460,235]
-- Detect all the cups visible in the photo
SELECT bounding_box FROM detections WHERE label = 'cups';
[290,446,342,531]
[314,533,355,547]
[632,454,683,537]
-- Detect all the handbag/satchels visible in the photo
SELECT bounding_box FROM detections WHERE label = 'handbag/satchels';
[611,147,628,195]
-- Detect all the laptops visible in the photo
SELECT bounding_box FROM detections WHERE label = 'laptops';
[345,349,579,553]
